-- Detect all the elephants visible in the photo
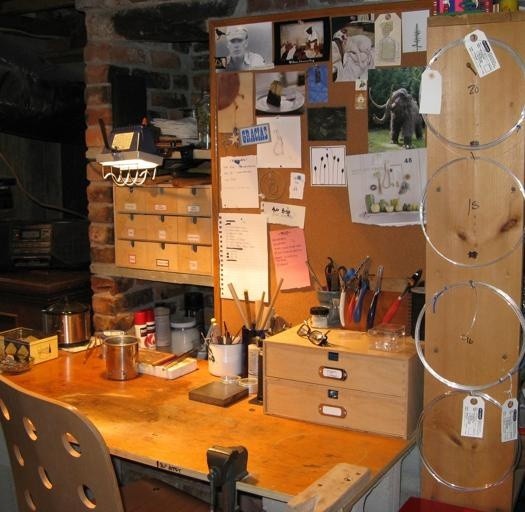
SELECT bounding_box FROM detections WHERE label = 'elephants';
[368,87,425,149]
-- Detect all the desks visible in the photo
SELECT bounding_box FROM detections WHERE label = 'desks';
[5,346,426,511]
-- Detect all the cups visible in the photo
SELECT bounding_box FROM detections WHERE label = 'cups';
[103,335,139,381]
[308,286,341,329]
[499,0,519,13]
[410,286,425,341]
[241,325,265,378]
[477,0,493,13]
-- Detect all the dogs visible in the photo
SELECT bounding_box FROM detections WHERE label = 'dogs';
[332,29,372,66]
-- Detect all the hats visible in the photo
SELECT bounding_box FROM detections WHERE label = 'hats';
[226,26,248,41]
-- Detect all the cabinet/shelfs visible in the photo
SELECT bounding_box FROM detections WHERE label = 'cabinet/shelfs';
[262,342,424,439]
[112,180,213,279]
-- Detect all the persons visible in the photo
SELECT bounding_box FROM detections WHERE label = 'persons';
[298,25,324,60]
[220,26,267,68]
[139,116,160,153]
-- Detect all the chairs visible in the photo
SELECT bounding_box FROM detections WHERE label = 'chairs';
[0,375,223,512]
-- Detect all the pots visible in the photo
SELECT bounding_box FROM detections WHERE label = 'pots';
[40,297,92,347]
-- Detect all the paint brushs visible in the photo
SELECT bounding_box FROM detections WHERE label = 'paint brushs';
[163,348,197,371]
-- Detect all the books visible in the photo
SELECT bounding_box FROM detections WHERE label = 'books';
[134,348,201,381]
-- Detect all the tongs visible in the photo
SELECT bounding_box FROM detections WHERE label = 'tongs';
[80,335,103,365]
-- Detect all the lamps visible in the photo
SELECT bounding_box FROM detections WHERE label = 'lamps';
[96,122,165,188]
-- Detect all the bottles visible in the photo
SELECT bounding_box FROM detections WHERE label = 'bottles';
[170,317,201,357]
[133,307,171,351]
[209,317,221,336]
[197,89,212,150]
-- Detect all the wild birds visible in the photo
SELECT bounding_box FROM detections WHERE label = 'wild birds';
[215,28,226,40]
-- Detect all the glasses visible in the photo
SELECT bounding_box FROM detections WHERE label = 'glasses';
[298,323,330,346]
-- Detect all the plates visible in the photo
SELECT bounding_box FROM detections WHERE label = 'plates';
[254,87,305,114]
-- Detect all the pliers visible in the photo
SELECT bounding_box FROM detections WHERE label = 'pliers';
[338,255,422,332]
